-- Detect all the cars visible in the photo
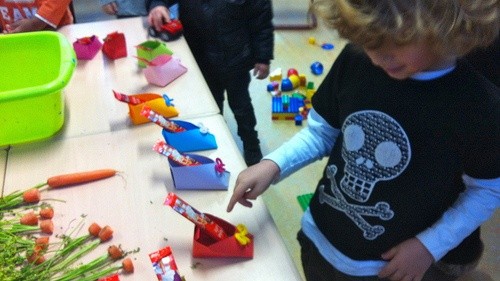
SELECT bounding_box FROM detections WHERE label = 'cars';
[148,17,183,42]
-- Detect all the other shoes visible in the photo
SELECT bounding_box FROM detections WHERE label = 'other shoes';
[240,131,263,167]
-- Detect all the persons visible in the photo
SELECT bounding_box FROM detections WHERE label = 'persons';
[0,0,74,34]
[227,0,500,281]
[144,0,275,167]
[99,0,178,20]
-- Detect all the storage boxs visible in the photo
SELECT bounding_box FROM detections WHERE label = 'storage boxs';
[1,30,76,148]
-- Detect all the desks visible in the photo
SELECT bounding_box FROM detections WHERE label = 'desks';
[3,113,301,280]
[10,15,220,148]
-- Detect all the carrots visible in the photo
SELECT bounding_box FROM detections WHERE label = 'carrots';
[89,222,133,273]
[47,169,118,187]
[20,188,54,264]
